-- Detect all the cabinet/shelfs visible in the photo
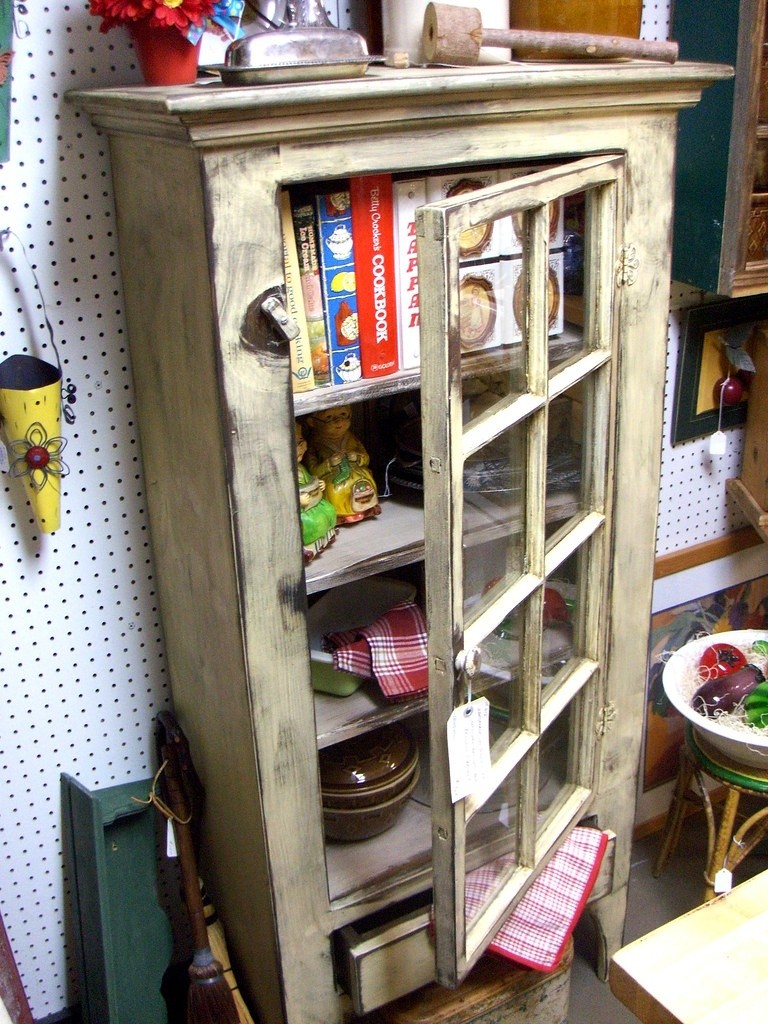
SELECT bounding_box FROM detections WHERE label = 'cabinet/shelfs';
[63,60,740,1024]
[668,0,768,298]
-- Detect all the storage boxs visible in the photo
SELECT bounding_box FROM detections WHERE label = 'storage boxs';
[377,932,573,1024]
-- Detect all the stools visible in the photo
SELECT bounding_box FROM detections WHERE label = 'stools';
[650,719,767,905]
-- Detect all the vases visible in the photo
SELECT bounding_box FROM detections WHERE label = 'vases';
[123,21,203,88]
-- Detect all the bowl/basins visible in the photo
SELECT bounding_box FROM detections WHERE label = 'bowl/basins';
[319,722,421,843]
[662,629,768,770]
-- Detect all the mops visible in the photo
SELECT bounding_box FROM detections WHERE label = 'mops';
[151,720,235,1024]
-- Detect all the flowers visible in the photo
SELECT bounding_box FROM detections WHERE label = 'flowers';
[86,0,223,35]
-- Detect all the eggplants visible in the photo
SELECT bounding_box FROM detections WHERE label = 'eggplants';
[693,663,765,716]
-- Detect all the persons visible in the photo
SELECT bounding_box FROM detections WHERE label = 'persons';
[292,420,339,566]
[306,404,383,525]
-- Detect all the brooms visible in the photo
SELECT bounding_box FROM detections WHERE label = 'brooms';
[156,711,253,1024]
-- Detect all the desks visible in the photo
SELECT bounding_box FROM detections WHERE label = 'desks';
[607,869,768,1024]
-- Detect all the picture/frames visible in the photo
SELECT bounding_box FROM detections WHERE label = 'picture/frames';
[671,294,768,446]
[632,523,768,844]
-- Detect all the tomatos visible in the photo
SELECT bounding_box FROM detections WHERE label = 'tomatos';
[700,643,747,680]
[481,576,502,596]
[543,588,567,624]
